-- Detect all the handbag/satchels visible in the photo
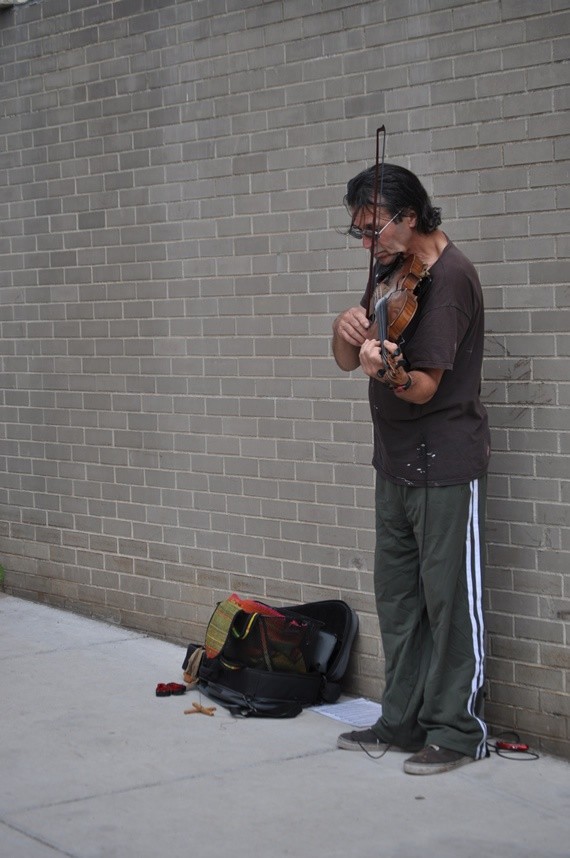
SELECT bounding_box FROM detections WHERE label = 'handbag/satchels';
[205,599,318,674]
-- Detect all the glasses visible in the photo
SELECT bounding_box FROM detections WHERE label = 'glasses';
[347,211,404,240]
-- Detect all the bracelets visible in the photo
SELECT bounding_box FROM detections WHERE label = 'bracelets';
[389,375,412,392]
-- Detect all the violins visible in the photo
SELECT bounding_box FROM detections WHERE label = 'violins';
[360,253,425,389]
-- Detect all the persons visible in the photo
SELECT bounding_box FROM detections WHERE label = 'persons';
[331,164,492,775]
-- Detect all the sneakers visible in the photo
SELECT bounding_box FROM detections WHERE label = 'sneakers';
[336,727,422,753]
[404,744,474,775]
